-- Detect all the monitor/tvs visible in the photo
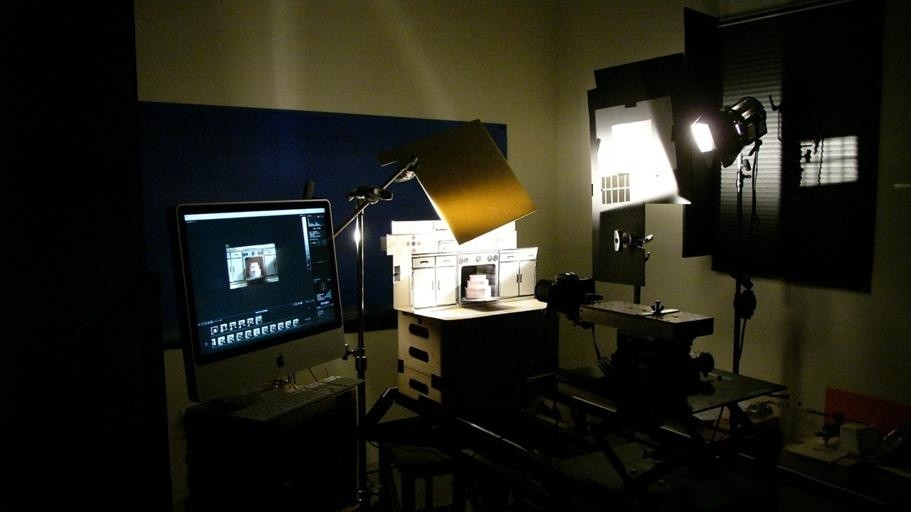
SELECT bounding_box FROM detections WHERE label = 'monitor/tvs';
[176,197,345,366]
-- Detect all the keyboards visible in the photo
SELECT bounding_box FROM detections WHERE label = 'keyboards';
[233,374,365,421]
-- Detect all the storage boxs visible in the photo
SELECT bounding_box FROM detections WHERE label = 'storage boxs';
[396,309,560,410]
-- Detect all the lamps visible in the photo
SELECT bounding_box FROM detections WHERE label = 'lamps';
[333,117,538,512]
[684,95,772,434]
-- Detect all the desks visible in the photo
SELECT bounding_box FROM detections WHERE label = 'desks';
[394,300,910,512]
